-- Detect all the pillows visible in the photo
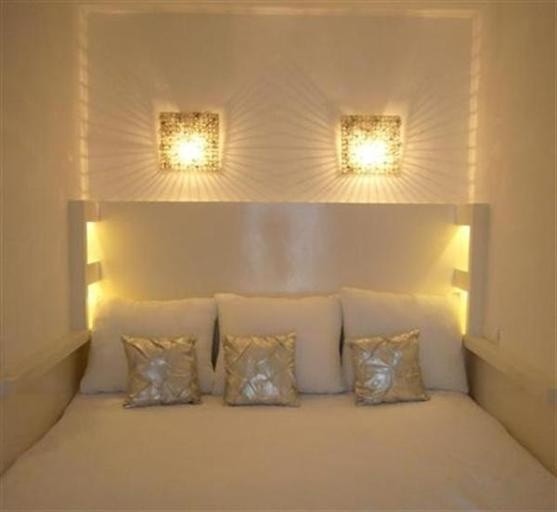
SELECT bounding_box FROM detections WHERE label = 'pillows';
[77,295,217,395]
[211,291,344,396]
[336,288,470,395]
[350,332,430,406]
[118,335,202,410]
[224,336,301,410]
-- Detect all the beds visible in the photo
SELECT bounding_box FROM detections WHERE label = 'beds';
[2,197,557,510]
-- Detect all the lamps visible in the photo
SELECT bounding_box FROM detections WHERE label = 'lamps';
[158,111,221,173]
[338,113,401,175]
